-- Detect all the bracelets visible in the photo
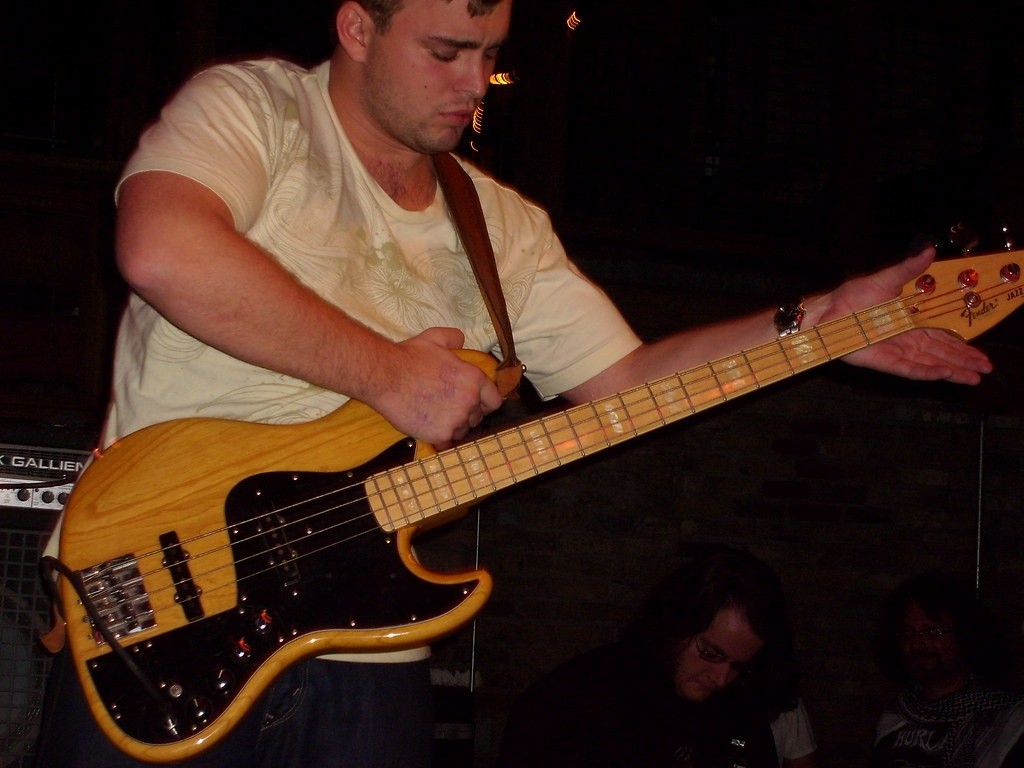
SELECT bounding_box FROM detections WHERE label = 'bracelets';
[774,296,805,337]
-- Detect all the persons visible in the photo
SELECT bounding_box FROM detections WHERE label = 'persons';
[868,571,1024,768]
[498,547,817,768]
[45,0,993,768]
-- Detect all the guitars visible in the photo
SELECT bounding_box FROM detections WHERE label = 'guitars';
[58,224,1024,768]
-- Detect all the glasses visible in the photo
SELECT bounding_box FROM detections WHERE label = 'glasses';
[695,635,747,671]
[895,624,955,641]
